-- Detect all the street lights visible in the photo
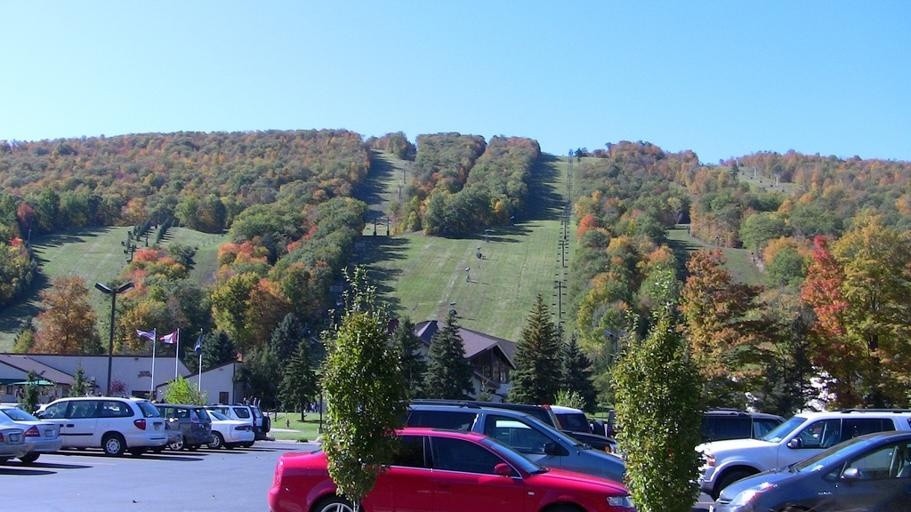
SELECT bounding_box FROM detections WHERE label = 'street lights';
[94,282,136,408]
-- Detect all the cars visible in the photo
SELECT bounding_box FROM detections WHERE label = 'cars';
[711,430,911,512]
[269,427,637,511]
[0,395,270,466]
[544,405,600,432]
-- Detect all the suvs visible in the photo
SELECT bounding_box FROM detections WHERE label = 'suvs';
[698,407,818,446]
[413,397,620,455]
[396,403,628,486]
[693,408,910,503]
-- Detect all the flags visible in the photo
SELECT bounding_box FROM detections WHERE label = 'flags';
[161,330,178,344]
[136,329,154,341]
[196,333,205,352]
